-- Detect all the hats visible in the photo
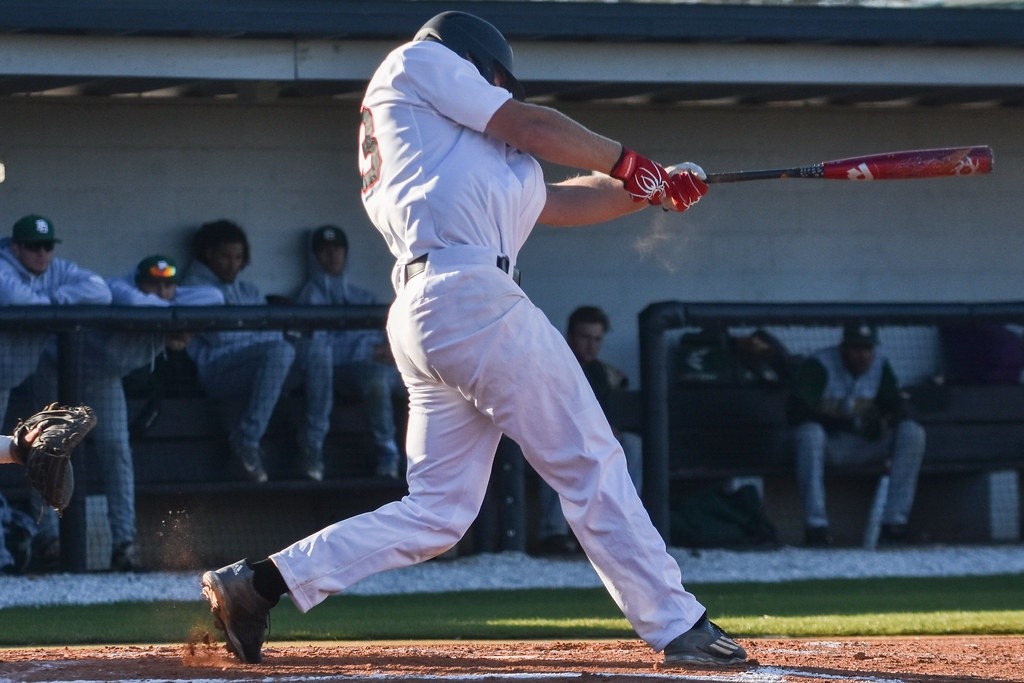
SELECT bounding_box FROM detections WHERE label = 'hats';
[312,224,349,250]
[13,215,61,242]
[843,323,880,345]
[136,254,179,281]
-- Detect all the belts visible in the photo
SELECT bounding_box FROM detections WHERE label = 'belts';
[403,258,522,284]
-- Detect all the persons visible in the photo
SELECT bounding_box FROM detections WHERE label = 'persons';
[675,321,793,538]
[789,316,927,551]
[281,223,407,476]
[196,7,751,663]
[171,218,306,485]
[535,308,655,557]
[0,214,116,573]
[66,254,223,570]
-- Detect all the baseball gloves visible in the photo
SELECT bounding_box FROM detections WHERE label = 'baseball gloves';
[12,402,96,512]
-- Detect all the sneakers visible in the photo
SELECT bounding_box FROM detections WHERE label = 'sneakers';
[665,613,749,671]
[201,556,271,664]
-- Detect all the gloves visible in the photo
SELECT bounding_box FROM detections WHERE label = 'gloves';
[660,161,707,212]
[609,144,667,205]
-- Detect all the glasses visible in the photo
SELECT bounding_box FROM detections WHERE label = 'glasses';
[147,264,177,278]
[22,241,55,252]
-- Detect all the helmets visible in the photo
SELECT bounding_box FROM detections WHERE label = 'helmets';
[412,12,518,90]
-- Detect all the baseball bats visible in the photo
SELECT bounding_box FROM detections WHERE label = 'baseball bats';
[703,144,993,182]
[862,419,901,551]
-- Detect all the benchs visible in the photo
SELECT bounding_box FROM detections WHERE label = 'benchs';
[0,382,1024,558]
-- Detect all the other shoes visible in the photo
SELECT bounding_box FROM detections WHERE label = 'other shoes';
[242,462,272,482]
[304,469,327,481]
[882,524,931,545]
[806,525,835,544]
